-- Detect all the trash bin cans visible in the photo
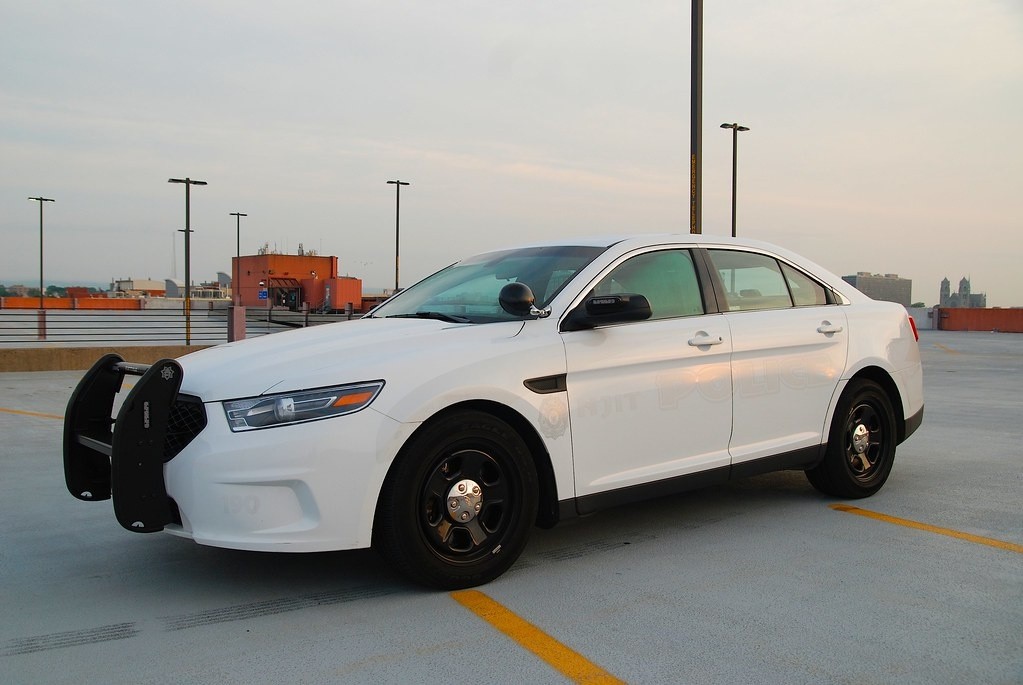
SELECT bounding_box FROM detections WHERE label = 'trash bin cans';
[208,302,213,311]
[302,301,310,314]
[345,302,354,315]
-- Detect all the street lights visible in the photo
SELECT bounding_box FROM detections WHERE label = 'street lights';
[720,121,750,294]
[26,196,55,309]
[228,212,248,305]
[387,179,410,296]
[168,176,207,345]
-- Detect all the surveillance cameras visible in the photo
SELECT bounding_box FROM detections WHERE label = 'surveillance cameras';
[259,282,264,286]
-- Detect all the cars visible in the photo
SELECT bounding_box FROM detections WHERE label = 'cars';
[60,233,929,592]
